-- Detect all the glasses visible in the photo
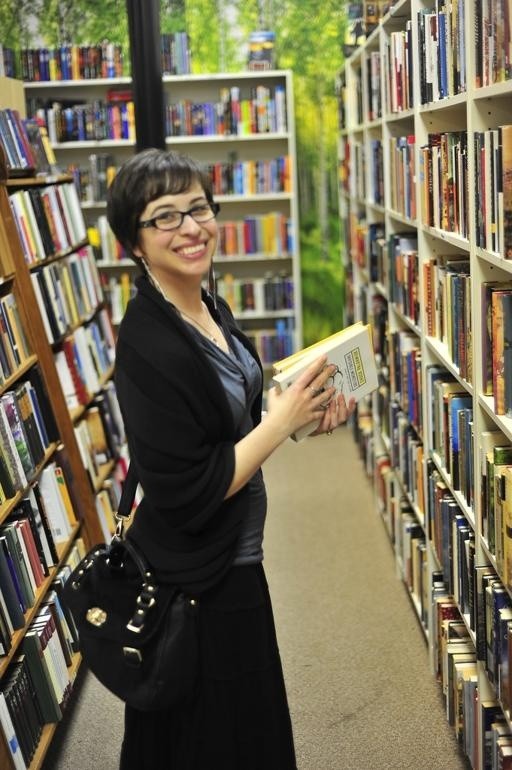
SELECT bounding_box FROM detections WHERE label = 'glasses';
[137,202,220,231]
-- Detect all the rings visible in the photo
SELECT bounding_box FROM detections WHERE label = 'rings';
[308,386,319,395]
[327,430,333,435]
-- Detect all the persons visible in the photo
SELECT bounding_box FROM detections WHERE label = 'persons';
[106,148,357,770]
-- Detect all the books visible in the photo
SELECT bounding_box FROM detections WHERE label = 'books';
[2,33,295,366]
[338,0,512,261]
[340,195,512,770]
[1,366,144,769]
[271,325,379,444]
[272,321,374,375]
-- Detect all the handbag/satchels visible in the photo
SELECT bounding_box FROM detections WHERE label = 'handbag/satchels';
[63,538,198,715]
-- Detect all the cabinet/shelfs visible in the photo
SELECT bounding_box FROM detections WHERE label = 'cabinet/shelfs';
[0,72,137,770]
[341,0,512,769]
[22,68,305,416]
